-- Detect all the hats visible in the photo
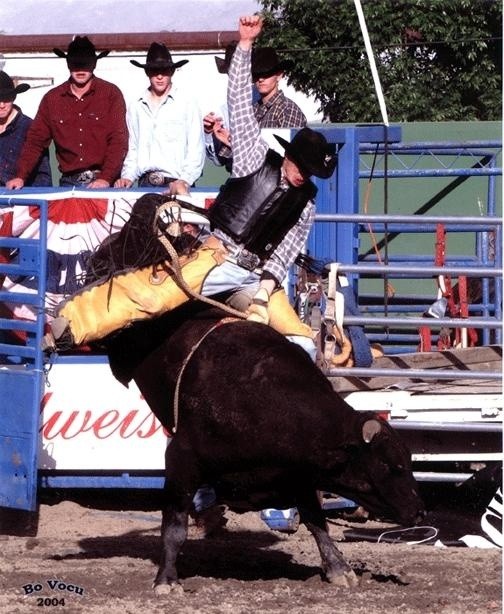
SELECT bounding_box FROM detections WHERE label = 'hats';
[129,41,190,71]
[0,70,31,100]
[251,46,296,82]
[53,34,111,61]
[272,129,338,180]
[214,38,239,75]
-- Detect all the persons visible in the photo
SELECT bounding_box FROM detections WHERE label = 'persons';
[4,36,128,190]
[46,15,318,364]
[213,47,308,170]
[0,70,53,187]
[204,39,263,167]
[113,42,206,195]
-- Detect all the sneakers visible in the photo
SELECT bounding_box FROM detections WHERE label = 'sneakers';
[41,318,73,352]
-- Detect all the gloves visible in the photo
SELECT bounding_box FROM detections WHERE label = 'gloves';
[247,287,271,326]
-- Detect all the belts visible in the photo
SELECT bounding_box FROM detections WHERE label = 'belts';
[214,231,265,271]
[138,172,179,186]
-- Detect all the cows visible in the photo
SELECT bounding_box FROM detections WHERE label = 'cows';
[81,189,428,598]
